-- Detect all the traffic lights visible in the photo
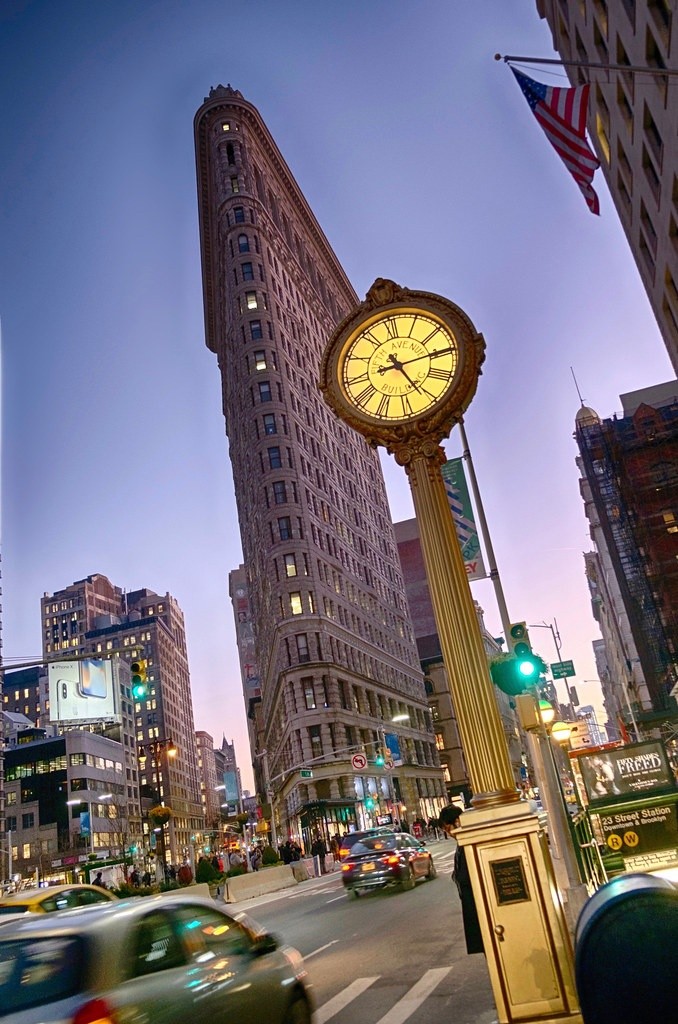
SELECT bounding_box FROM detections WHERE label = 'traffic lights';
[131,659,149,697]
[376,747,384,765]
[507,620,540,688]
[366,793,373,808]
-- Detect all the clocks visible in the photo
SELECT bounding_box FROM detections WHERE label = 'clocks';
[318,277,484,461]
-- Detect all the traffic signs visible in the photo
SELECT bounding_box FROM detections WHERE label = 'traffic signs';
[551,660,575,680]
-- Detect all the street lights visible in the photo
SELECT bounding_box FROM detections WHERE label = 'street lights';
[534,699,588,888]
[378,711,412,825]
[551,721,605,881]
[66,793,113,853]
[138,736,177,880]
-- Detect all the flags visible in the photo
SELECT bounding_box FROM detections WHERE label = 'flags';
[505,60,603,218]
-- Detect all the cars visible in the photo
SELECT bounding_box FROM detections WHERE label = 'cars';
[341,832,437,901]
[0,892,317,1024]
[339,826,402,864]
[0,884,120,927]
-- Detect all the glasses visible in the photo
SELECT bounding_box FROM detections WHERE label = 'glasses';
[447,824,452,835]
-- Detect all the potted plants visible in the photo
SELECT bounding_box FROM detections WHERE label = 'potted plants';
[259,844,284,871]
[196,859,222,899]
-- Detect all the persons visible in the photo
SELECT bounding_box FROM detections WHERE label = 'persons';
[394,816,448,840]
[311,839,319,878]
[91,872,107,891]
[330,832,347,863]
[310,834,330,875]
[109,839,306,901]
[516,781,540,800]
[439,802,488,960]
[589,755,620,797]
